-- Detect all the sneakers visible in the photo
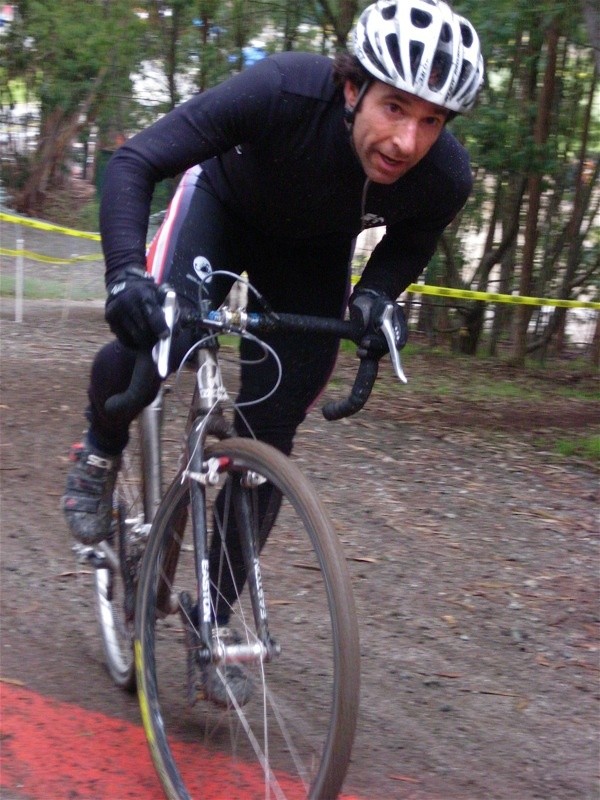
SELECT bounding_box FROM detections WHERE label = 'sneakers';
[62,435,123,546]
[200,626,251,707]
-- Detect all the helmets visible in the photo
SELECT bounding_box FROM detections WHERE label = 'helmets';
[354,0,484,113]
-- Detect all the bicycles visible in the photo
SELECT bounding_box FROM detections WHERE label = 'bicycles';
[74,264,410,800]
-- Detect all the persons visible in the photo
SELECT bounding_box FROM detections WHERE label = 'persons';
[58,0,485,709]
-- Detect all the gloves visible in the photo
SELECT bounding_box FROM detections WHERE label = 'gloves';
[348,286,408,359]
[105,266,170,355]
